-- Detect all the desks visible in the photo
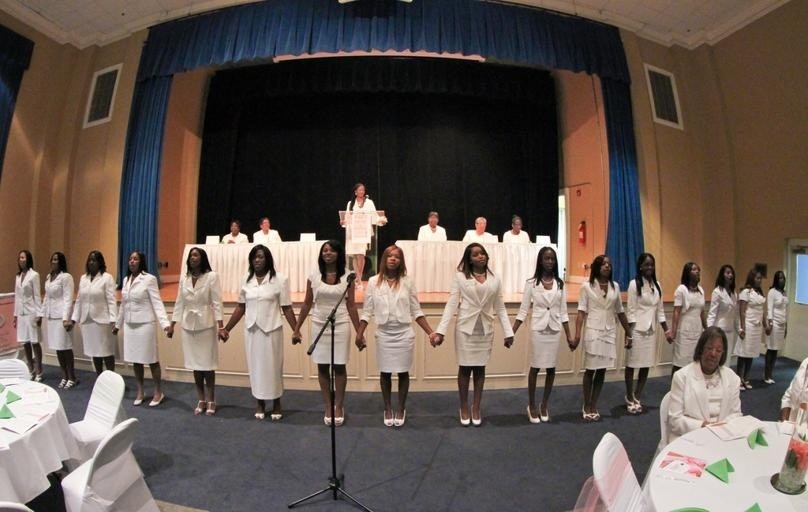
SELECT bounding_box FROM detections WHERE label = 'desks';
[394,240,557,293]
[179,240,330,293]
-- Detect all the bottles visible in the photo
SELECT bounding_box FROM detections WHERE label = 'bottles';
[775,393,807,493]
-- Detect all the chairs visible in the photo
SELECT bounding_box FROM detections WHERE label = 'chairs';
[206,235,219,244]
[0,358,162,511]
[300,233,316,241]
[536,235,551,244]
[573,392,807,512]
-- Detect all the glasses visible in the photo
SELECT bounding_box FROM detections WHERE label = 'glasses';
[705,345,724,356]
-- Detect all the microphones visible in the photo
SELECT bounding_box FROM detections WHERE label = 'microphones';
[347,273,357,290]
[365,195,369,210]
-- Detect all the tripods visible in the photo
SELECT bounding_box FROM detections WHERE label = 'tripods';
[289,288,373,512]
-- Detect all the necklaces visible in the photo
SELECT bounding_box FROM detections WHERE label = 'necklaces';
[387,277,396,282]
[326,272,337,275]
[600,283,607,287]
[256,275,265,280]
[542,278,553,284]
[473,271,486,277]
[193,272,200,278]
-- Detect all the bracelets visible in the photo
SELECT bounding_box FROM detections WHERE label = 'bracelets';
[219,327,225,330]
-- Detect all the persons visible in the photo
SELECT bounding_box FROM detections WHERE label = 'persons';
[222,217,282,246]
[221,245,303,421]
[340,184,388,289]
[355,245,440,427]
[417,211,531,245]
[430,243,514,426]
[625,253,808,444]
[504,247,574,424]
[167,247,229,416]
[112,250,171,407]
[14,249,117,391]
[292,240,366,427]
[571,255,633,422]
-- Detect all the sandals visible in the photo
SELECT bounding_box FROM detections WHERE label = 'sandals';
[194,399,346,425]
[740,378,777,391]
[381,393,644,426]
[27,369,163,407]
